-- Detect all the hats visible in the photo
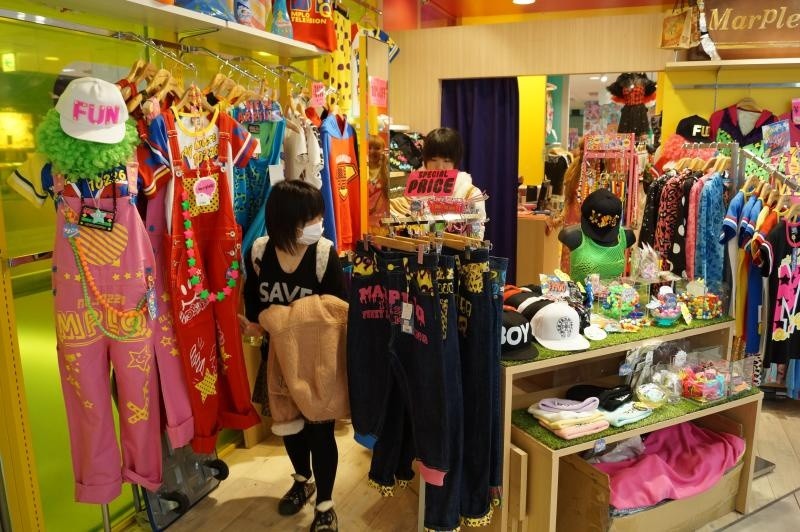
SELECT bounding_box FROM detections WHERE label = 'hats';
[581,187,624,249]
[54,76,129,144]
[498,284,591,360]
[527,383,653,440]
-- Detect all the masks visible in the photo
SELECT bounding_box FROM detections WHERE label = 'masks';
[295,219,324,245]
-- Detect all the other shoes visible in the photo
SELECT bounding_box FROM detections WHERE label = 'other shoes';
[309,499,339,532]
[277,472,316,516]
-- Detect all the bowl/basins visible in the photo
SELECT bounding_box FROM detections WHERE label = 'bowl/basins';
[655,312,681,327]
[524,203,537,210]
[636,389,667,409]
[675,278,730,319]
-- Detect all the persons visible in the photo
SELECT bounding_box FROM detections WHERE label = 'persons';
[559,188,637,288]
[237,179,348,532]
[420,126,486,241]
[543,157,581,276]
